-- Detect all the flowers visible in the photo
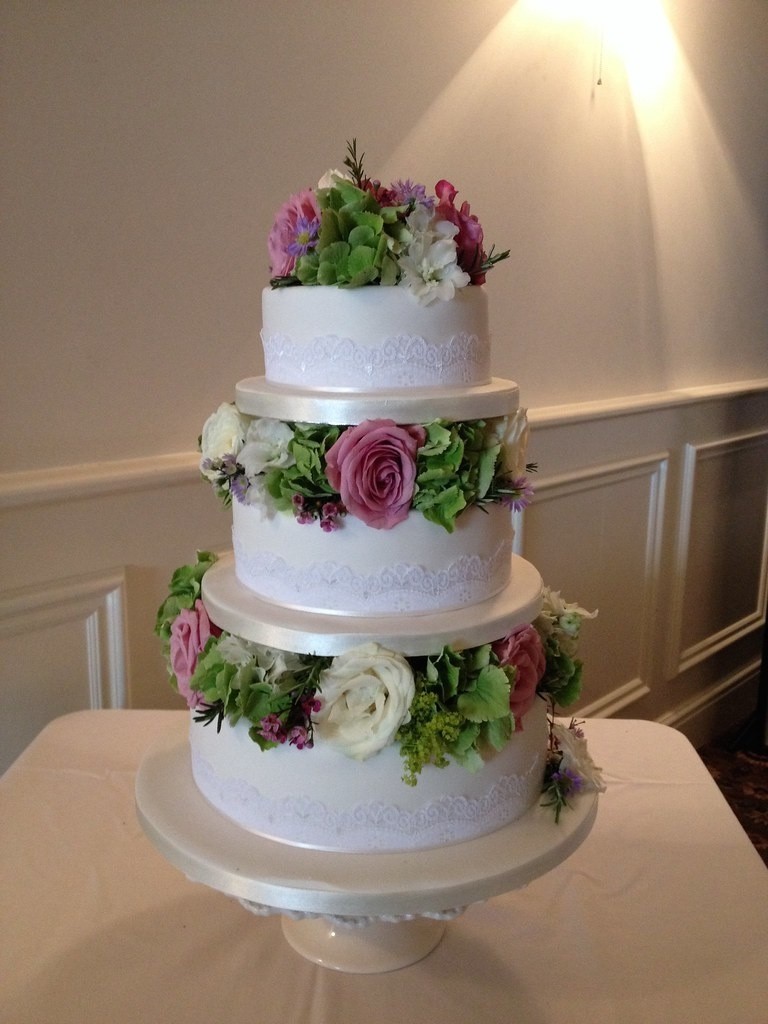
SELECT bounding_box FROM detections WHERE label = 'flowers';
[266,138,512,308]
[154,549,608,825]
[196,401,542,533]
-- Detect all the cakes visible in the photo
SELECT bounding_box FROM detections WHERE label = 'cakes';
[154,139,602,854]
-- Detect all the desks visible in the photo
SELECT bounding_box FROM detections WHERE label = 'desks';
[1,711,767,1023]
